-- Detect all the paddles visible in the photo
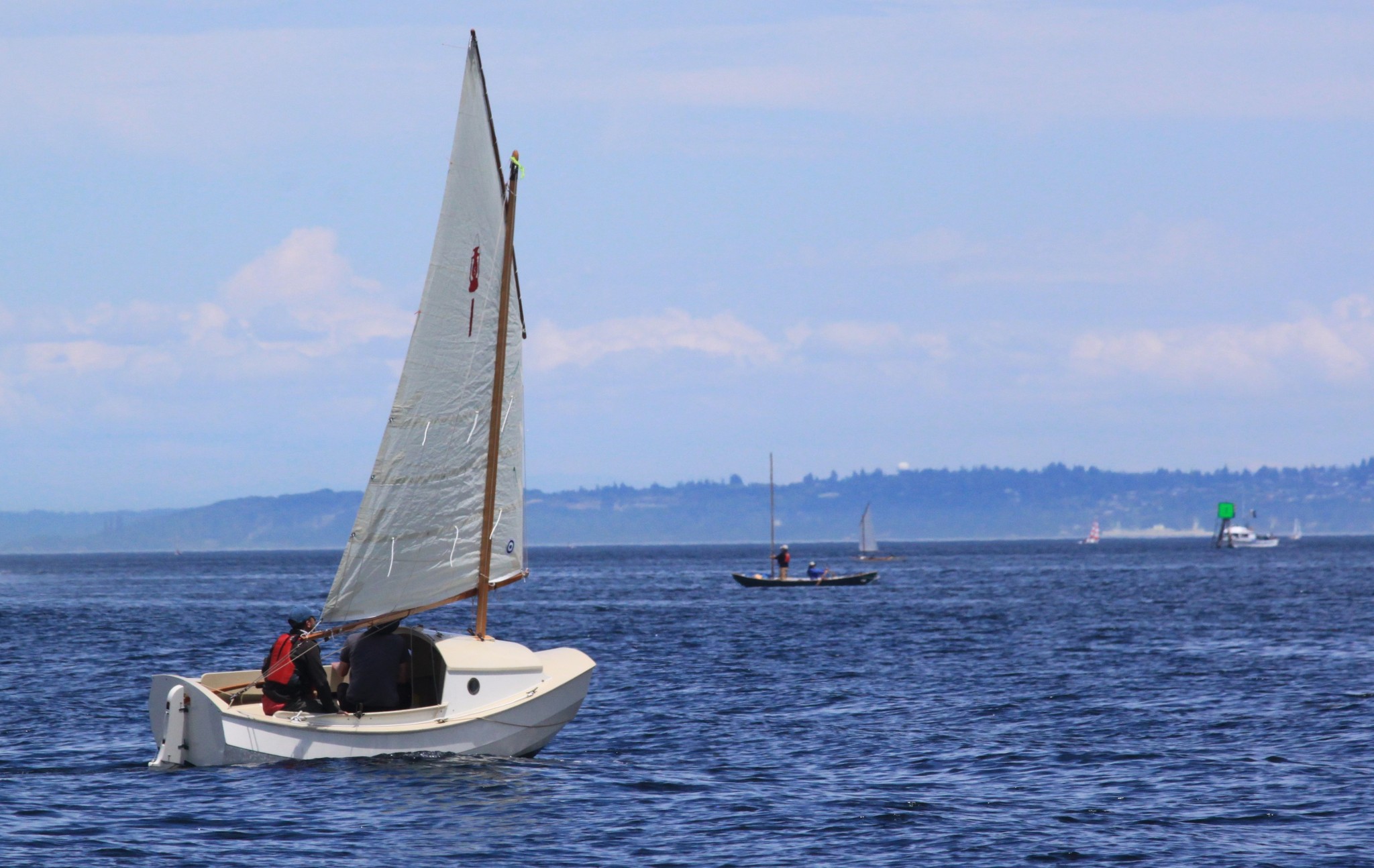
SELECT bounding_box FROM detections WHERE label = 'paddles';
[816,570,828,585]
[827,568,835,576]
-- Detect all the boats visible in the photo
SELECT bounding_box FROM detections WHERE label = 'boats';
[1209,502,1282,551]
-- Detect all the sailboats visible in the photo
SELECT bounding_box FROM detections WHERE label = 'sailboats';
[1287,517,1304,541]
[146,27,599,776]
[846,502,899,562]
[731,449,878,589]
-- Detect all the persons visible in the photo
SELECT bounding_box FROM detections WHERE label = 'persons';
[263,605,337,717]
[807,561,833,578]
[769,545,790,580]
[331,619,413,713]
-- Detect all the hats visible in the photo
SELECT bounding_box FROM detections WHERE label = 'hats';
[809,561,815,566]
[780,545,789,550]
[288,604,321,626]
